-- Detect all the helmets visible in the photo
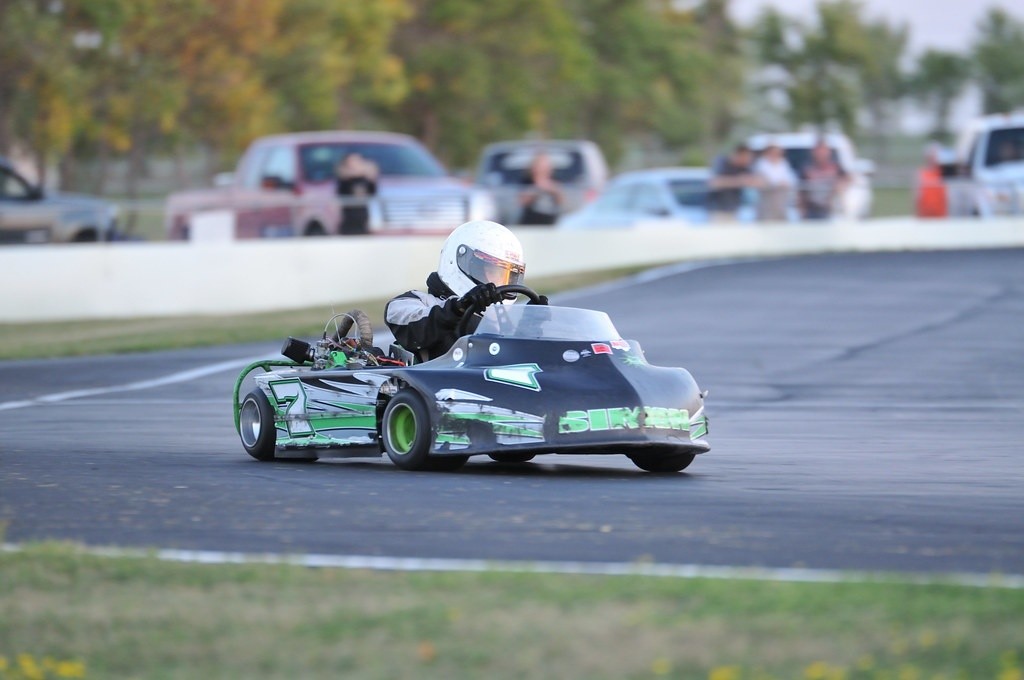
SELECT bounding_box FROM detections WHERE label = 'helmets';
[437,220,526,321]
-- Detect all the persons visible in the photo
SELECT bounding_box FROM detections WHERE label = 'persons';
[336,152,381,236]
[708,143,851,220]
[383,221,551,365]
[519,154,567,224]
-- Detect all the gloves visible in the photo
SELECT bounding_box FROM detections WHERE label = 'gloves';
[522,294,551,323]
[460,282,502,314]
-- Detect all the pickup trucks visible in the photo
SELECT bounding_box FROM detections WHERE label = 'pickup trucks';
[162,130,499,239]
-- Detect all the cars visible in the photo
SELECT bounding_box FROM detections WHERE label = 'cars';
[551,164,802,223]
[745,134,879,221]
[477,137,610,226]
[0,159,121,246]
[936,110,1024,215]
